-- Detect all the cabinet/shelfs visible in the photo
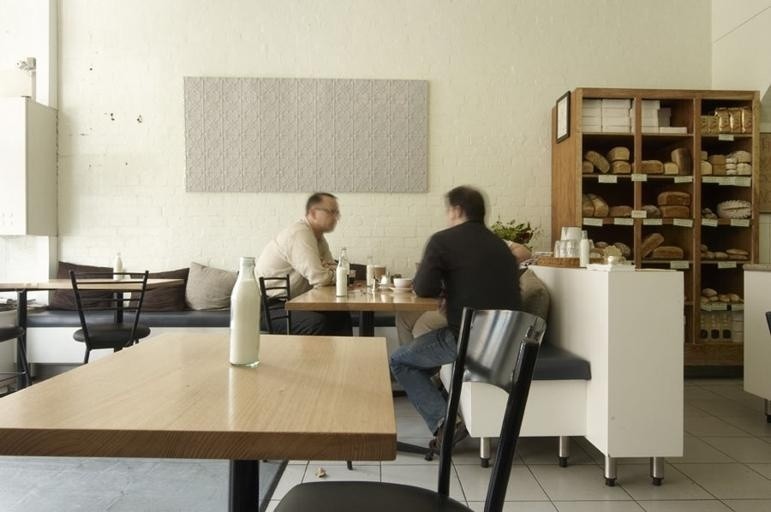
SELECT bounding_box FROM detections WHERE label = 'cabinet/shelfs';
[550,88,758,369]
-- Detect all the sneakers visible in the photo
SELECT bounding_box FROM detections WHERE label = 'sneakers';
[429,422,468,455]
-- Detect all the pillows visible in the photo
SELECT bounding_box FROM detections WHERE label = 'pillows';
[184,259,238,311]
[131,267,189,312]
[517,270,552,318]
[52,261,125,315]
[379,258,420,281]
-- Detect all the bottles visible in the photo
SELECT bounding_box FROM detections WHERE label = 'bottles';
[230,256,262,367]
[367,256,375,286]
[341,248,350,286]
[336,256,347,296]
[114,251,122,282]
[579,231,590,267]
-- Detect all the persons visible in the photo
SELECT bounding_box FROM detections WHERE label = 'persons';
[501,237,530,263]
[388,185,521,455]
[255,191,341,336]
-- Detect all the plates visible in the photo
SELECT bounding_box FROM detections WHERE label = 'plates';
[390,287,412,292]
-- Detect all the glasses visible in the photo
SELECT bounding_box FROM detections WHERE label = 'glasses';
[315,208,339,215]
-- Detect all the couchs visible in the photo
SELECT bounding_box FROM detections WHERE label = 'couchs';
[15,263,259,375]
[371,261,685,489]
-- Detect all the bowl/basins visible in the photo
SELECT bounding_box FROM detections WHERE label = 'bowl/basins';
[394,279,412,288]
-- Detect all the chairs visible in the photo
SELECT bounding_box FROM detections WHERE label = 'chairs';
[67,269,155,366]
[275,308,547,512]
[259,274,291,338]
[0,326,32,396]
